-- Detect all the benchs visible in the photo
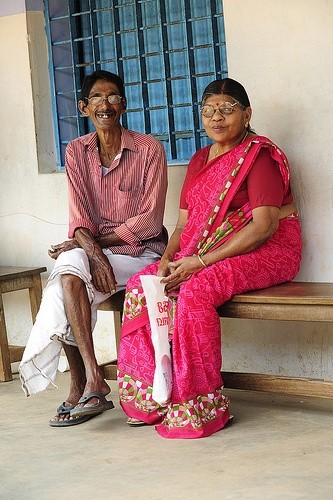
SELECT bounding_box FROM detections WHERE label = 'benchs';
[0,266,48,381]
[92,278,333,399]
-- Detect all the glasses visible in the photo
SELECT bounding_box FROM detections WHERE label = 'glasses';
[200,101,238,118]
[84,95,122,106]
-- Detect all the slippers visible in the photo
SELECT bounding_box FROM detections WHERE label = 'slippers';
[69,392,115,418]
[48,402,103,426]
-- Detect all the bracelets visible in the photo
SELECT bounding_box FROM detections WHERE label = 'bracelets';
[198,255,207,269]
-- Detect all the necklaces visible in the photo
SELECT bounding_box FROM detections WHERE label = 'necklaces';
[214,131,248,159]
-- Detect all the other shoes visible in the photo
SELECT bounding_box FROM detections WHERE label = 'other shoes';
[126,417,147,427]
[223,415,234,426]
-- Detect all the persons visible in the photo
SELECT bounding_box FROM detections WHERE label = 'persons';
[18,70,169,427]
[118,78,302,438]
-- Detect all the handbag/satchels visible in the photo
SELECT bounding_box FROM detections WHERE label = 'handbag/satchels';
[139,275,177,407]
